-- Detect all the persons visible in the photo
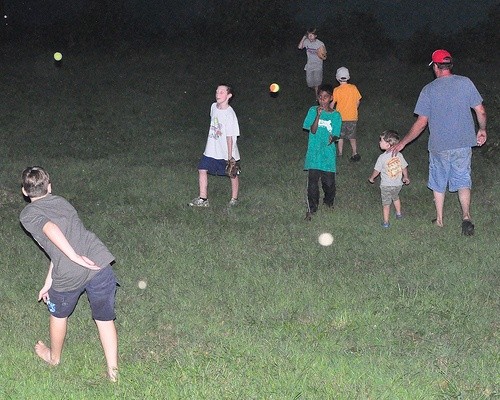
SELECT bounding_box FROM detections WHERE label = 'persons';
[18,166,122,383]
[328,66,362,162]
[298,27,327,102]
[302,83,342,223]
[385,50,488,236]
[368,130,410,227]
[187,84,241,208]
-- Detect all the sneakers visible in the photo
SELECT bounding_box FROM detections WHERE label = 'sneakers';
[351,153,360,161]
[226,198,239,208]
[187,197,209,207]
[381,222,388,227]
[395,215,401,220]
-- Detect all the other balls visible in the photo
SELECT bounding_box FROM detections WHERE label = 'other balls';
[53,51,63,61]
[138,282,146,289]
[270,83,280,93]
[319,232,336,247]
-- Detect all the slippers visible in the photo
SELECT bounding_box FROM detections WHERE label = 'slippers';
[462,219,474,236]
[432,218,436,223]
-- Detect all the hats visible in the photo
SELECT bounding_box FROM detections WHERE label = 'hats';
[336,67,350,81]
[428,49,453,70]
